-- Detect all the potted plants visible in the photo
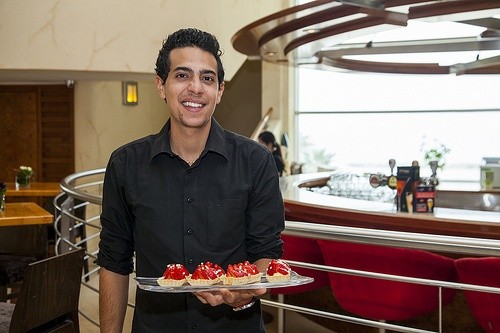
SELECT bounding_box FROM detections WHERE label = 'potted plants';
[425,145,450,176]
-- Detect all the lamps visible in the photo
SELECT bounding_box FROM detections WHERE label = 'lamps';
[122,81,138,105]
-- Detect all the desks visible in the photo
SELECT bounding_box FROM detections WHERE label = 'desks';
[4,182,62,196]
[0,203,54,226]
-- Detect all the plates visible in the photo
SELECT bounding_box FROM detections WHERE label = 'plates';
[133,272,314,293]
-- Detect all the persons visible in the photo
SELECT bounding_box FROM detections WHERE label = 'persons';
[96,28,286,333]
[258,131,287,195]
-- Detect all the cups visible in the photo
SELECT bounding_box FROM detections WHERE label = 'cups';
[329,173,398,197]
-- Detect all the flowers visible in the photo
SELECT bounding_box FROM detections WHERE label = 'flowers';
[17,165,32,184]
[0,183,6,206]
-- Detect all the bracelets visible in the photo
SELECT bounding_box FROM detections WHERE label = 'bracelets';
[233,298,257,312]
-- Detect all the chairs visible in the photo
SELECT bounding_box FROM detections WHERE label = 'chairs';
[455,257,500,333]
[315,239,461,333]
[0,248,85,333]
[271,234,327,333]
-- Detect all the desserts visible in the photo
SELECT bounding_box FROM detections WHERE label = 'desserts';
[157,258,292,286]
